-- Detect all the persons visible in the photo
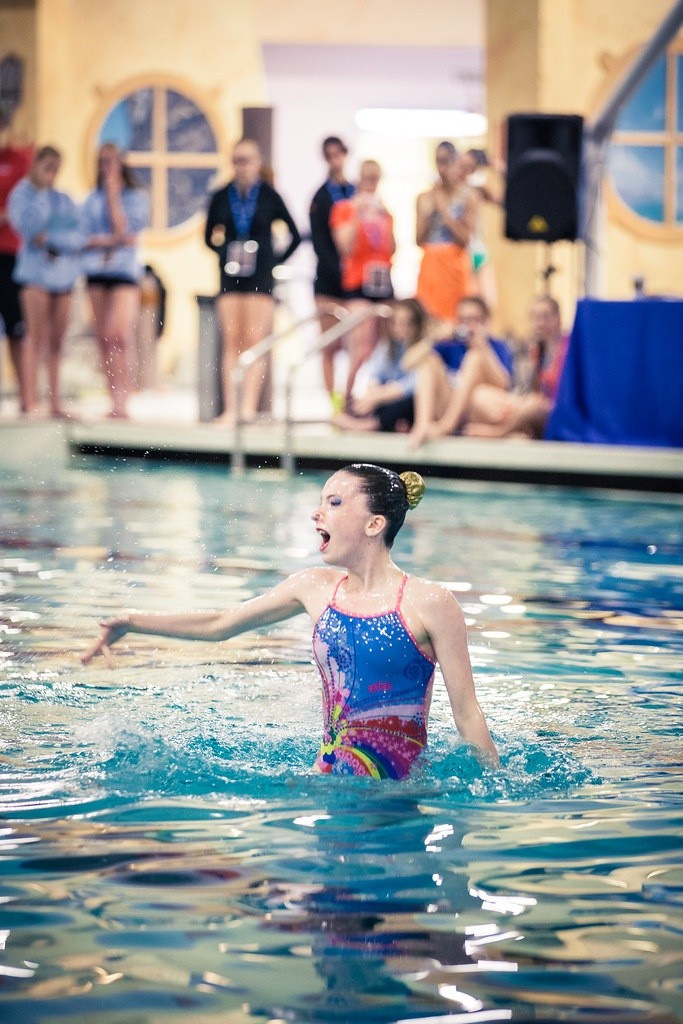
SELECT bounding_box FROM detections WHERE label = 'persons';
[462,296,569,439]
[329,160,396,417]
[6,147,86,420]
[309,136,355,413]
[205,139,300,424]
[398,298,514,436]
[78,143,152,420]
[415,141,493,321]
[0,111,35,413]
[81,463,501,780]
[352,299,428,433]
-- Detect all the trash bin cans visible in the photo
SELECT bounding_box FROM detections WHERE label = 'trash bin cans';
[194,295,284,422]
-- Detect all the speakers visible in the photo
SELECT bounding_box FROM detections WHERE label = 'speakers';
[504,114,582,242]
